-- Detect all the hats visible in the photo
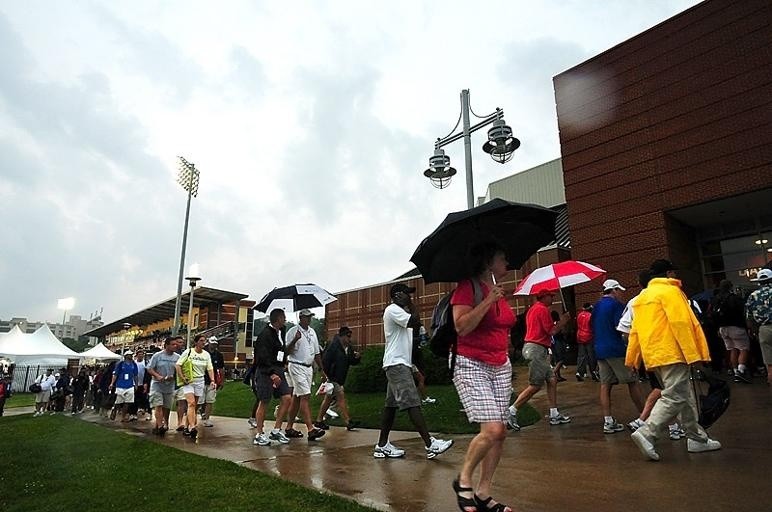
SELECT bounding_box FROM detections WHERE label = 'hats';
[603,278,626,293]
[299,309,315,316]
[54,373,61,376]
[124,350,134,356]
[756,269,772,280]
[209,335,219,344]
[339,326,352,336]
[390,284,416,295]
[536,289,557,298]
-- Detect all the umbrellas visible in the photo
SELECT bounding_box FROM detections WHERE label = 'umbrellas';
[251,281,339,316]
[410,198,561,287]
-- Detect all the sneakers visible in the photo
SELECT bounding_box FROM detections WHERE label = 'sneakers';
[550,364,600,382]
[505,409,520,431]
[602,413,722,460]
[728,362,769,384]
[248,405,361,446]
[422,397,436,405]
[33,407,214,441]
[425,437,455,458]
[373,442,406,458]
[549,413,574,424]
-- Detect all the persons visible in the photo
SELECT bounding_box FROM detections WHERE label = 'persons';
[746,270,770,387]
[313,325,363,432]
[590,279,645,434]
[575,302,599,383]
[0,355,18,418]
[624,259,721,460]
[447,244,519,511]
[253,308,303,446]
[373,280,453,460]
[548,309,567,382]
[284,308,330,443]
[509,288,573,431]
[242,358,266,429]
[617,269,730,438]
[28,333,229,445]
[717,278,749,383]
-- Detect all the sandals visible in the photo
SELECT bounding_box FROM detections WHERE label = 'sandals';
[473,491,513,512]
[452,472,478,511]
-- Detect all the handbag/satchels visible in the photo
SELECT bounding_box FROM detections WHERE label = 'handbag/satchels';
[695,363,730,427]
[175,360,193,389]
[30,383,42,392]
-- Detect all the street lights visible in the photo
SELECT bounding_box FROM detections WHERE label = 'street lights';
[185,277,202,349]
[422,89,521,211]
[119,322,132,356]
[172,155,200,340]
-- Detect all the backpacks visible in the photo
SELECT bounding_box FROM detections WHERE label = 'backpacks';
[429,276,483,380]
